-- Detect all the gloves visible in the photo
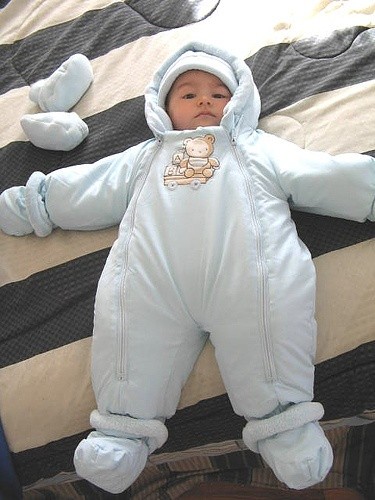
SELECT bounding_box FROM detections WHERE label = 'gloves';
[0,171,52,238]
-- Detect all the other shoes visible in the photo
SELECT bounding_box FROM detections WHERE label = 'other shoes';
[73,409,168,494]
[20,111,89,151]
[29,53,94,112]
[242,402,334,490]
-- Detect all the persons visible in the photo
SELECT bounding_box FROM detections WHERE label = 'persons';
[0,40,375,494]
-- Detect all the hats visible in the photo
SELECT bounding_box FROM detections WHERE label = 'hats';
[156,50,238,108]
